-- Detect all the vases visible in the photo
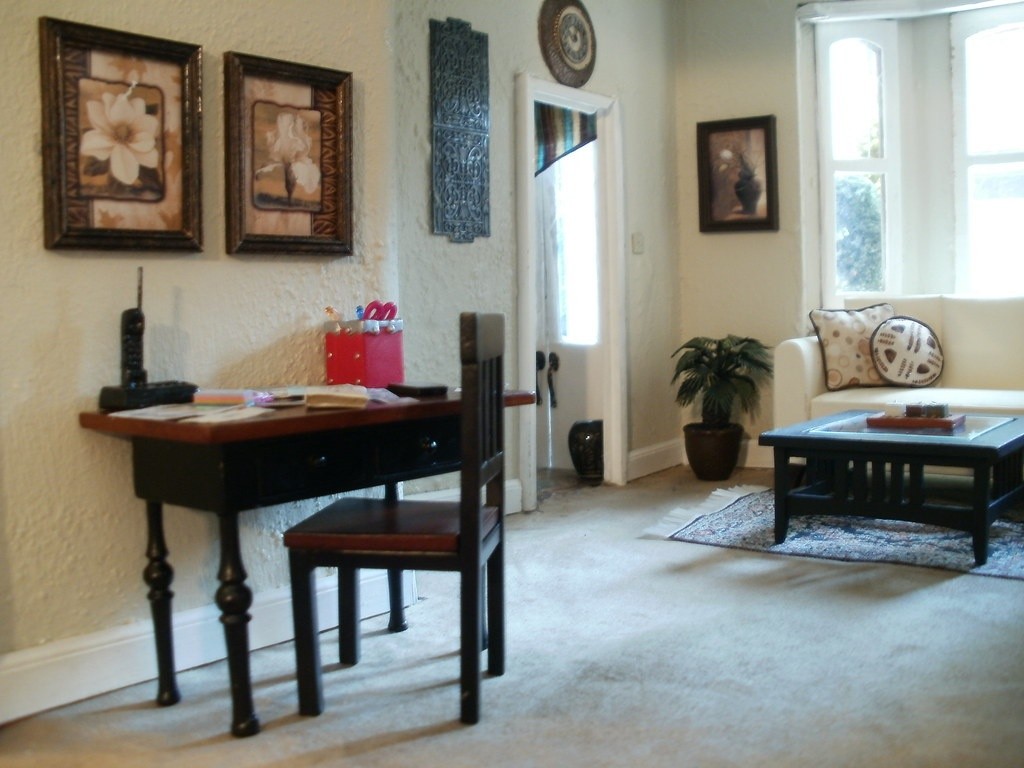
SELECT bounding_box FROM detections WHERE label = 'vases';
[568,419,604,482]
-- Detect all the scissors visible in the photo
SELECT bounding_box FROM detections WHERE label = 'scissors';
[363,301,397,319]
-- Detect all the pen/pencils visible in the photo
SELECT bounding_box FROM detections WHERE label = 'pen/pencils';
[239,395,273,410]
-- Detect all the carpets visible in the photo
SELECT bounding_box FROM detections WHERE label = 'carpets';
[638,483,1024,581]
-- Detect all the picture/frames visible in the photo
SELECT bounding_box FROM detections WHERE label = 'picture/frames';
[39,15,204,254]
[695,113,778,230]
[224,51,352,254]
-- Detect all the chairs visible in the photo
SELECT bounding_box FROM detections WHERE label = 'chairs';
[284,308,505,725]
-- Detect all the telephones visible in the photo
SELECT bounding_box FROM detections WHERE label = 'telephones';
[98,267,201,409]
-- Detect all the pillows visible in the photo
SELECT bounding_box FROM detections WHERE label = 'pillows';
[870,316,944,387]
[809,304,896,391]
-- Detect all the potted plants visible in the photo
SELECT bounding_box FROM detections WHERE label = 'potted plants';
[670,333,773,482]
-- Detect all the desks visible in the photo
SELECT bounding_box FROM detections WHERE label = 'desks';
[80,389,537,735]
[759,409,1024,565]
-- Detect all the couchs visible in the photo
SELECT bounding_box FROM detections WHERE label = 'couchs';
[772,293,1024,494]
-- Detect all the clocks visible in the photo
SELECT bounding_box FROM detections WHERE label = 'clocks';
[540,0,596,88]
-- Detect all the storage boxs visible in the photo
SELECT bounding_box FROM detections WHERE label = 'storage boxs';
[326,319,404,387]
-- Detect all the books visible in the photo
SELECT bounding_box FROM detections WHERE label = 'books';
[194,383,369,410]
